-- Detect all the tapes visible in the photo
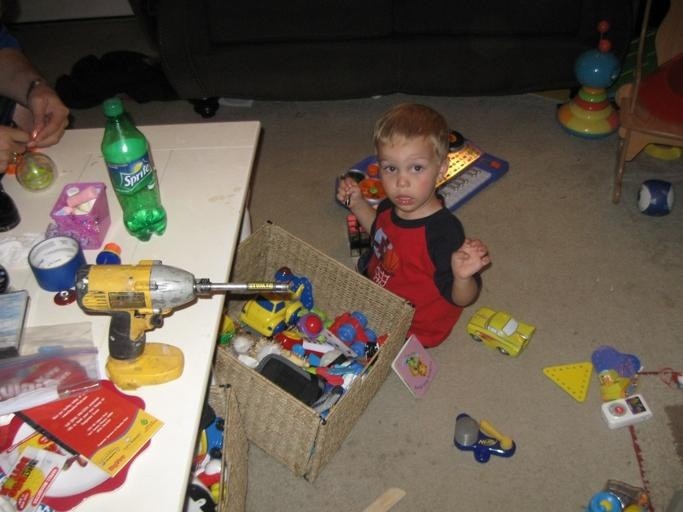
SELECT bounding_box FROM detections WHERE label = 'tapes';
[28,236,86,292]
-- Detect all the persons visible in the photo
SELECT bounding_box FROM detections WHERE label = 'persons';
[337,102,492,349]
[0,23,70,176]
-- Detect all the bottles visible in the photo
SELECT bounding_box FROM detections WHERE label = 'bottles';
[98,96,168,242]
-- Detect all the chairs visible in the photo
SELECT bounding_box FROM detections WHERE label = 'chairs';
[613,0,683,204]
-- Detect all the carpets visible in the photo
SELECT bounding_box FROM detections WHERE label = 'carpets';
[624,372,682,512]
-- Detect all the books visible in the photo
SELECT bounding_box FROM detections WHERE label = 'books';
[392,334,437,399]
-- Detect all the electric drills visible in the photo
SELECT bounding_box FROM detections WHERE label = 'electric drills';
[75,259,290,391]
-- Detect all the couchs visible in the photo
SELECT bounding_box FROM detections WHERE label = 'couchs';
[157,0,636,120]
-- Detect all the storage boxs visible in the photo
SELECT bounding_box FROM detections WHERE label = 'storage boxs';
[181,382,250,512]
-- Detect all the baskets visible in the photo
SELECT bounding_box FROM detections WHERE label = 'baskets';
[206,383,248,512]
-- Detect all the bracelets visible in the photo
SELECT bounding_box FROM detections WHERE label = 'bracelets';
[25,77,47,99]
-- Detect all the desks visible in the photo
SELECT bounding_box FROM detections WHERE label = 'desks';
[1,119,261,512]
